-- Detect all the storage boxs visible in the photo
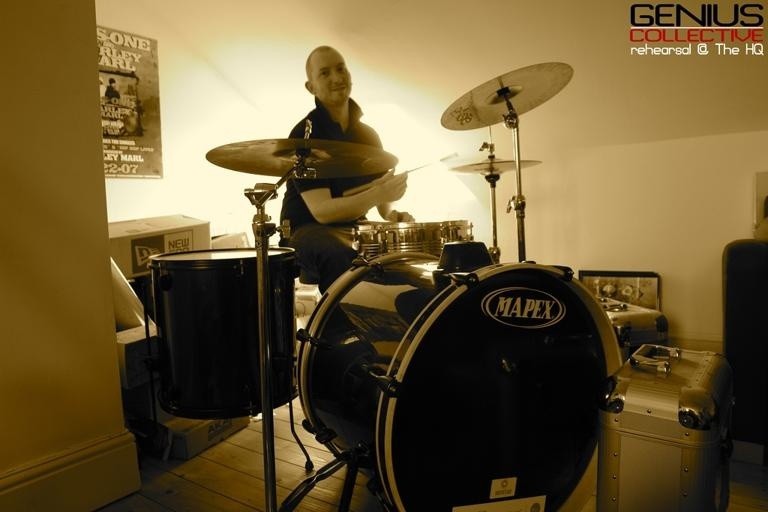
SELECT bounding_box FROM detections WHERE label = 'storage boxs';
[108,215,211,279]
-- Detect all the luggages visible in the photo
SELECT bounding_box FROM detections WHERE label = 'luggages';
[595,344,737,512]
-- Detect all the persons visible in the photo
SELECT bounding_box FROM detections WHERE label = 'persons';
[278,44,416,297]
[104,78,121,104]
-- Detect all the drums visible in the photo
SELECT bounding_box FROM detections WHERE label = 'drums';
[295,242,622,512]
[352,218,474,265]
[147,246,299,418]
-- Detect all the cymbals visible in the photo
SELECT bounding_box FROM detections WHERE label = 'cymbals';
[206,139,398,179]
[449,159,542,174]
[441,62,573,131]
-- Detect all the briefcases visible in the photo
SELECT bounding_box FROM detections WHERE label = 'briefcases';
[594,296,669,364]
